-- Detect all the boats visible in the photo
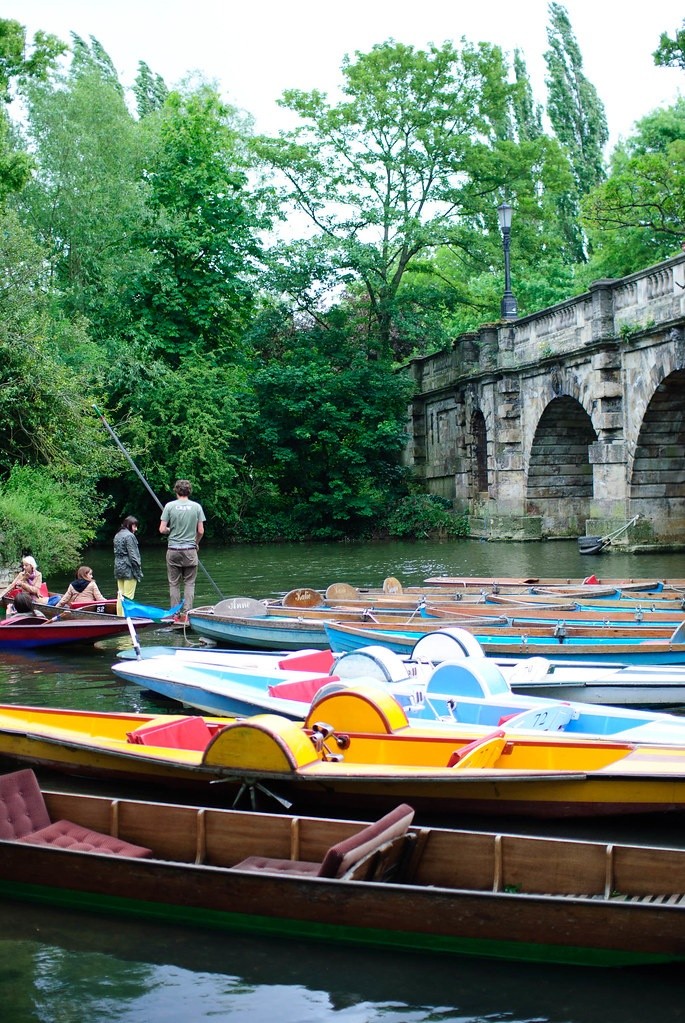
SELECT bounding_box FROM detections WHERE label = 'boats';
[113,646,685,747]
[0,688,685,819]
[1,596,154,651]
[191,575,685,710]
[0,768,685,972]
[0,586,189,633]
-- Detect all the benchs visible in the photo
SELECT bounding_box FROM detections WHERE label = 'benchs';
[9,582,50,599]
[497,700,579,731]
[230,804,416,879]
[0,768,153,859]
[280,649,334,674]
[270,675,340,702]
[126,716,213,751]
[448,729,507,769]
[70,598,118,615]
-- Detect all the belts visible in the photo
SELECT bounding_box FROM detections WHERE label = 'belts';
[170,547,196,550]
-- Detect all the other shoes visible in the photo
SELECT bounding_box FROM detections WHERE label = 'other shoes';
[172,612,181,619]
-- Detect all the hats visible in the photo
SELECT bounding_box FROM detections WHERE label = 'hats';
[173,480,193,494]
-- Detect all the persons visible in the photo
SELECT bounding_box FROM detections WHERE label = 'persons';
[56,566,108,608]
[0,557,61,606]
[159,480,206,622]
[6,593,46,620]
[114,516,144,616]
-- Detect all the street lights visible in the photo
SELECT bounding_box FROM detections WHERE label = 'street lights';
[495,200,520,318]
